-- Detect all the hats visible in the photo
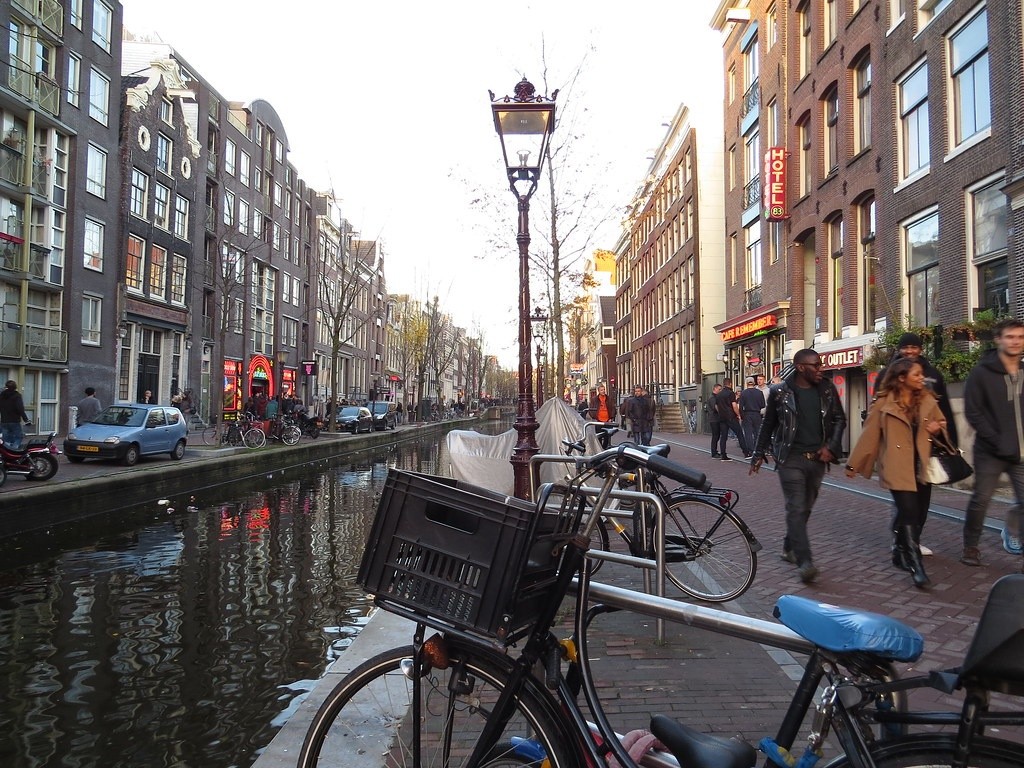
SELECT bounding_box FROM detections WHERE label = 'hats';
[746,377,755,385]
[85,387,95,393]
[896,333,921,350]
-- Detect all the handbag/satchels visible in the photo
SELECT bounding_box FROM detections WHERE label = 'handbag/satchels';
[925,425,974,484]
[190,405,196,414]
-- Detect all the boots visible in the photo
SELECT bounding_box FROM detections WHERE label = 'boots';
[893,523,930,590]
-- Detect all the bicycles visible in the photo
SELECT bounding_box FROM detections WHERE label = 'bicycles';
[202,412,302,450]
[297,441,1024,768]
[536,422,762,604]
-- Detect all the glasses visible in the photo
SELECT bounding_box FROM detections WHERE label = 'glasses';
[802,361,822,370]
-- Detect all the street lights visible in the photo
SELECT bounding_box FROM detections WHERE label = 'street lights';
[530,307,549,411]
[487,76,561,497]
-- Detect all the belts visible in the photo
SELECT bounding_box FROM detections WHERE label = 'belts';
[803,451,818,459]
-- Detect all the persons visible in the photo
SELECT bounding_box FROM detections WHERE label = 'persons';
[76,388,103,428]
[620,384,656,445]
[706,374,782,461]
[396,402,417,424]
[959,319,1024,567]
[243,392,307,440]
[589,384,617,450]
[842,358,947,589]
[578,400,588,420]
[141,390,157,404]
[324,398,368,417]
[481,393,515,407]
[0,380,29,448]
[749,349,846,583]
[872,333,974,555]
[172,389,194,436]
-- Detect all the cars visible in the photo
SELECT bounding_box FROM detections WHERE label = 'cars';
[63,403,188,466]
[324,405,373,434]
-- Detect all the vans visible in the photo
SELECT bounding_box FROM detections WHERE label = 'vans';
[363,401,397,431]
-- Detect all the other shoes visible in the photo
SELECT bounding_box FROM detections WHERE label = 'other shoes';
[712,453,721,459]
[744,450,773,459]
[721,453,733,461]
[627,431,636,438]
[779,550,821,581]
[919,543,933,555]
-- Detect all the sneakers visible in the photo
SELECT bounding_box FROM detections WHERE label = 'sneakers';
[1000,527,1024,556]
[960,547,982,568]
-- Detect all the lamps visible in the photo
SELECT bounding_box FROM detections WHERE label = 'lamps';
[745,344,766,366]
[723,350,740,371]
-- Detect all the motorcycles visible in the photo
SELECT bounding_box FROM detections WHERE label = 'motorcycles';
[296,409,323,439]
[0,432,63,487]
